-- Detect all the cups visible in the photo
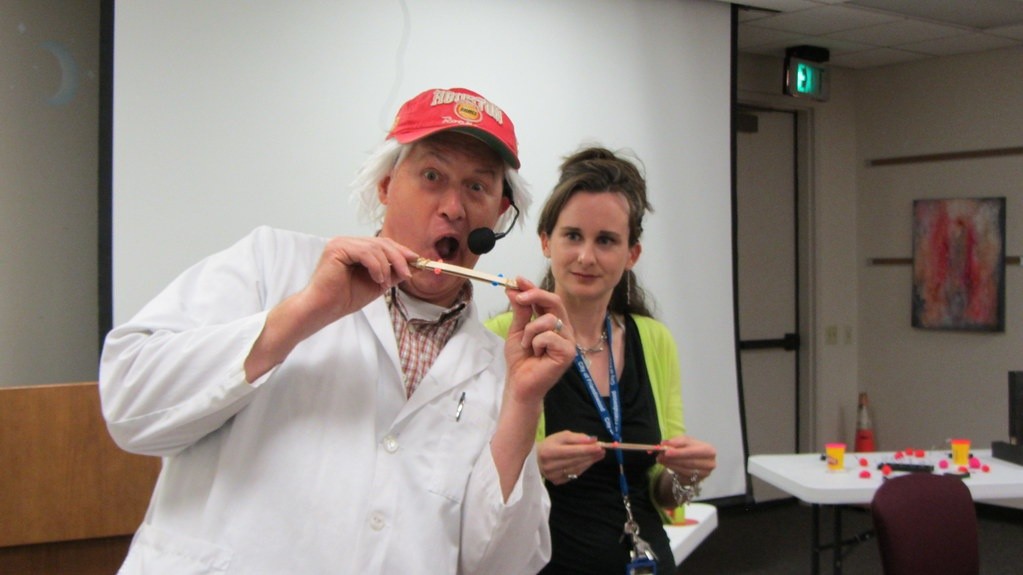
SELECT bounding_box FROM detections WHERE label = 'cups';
[824,442,847,471]
[950,437,972,466]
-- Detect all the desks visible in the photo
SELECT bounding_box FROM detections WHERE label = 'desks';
[663,502,719,569]
[746,449,1023,575]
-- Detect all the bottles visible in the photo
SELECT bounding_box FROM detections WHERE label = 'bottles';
[855,392,874,452]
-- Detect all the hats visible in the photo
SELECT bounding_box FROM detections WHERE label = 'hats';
[386,88,521,171]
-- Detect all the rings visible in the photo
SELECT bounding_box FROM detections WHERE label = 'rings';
[553,320,563,333]
[568,474,577,479]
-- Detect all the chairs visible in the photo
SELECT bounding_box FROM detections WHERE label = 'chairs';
[870,472,980,575]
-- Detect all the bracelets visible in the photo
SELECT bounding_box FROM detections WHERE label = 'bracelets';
[667,468,700,503]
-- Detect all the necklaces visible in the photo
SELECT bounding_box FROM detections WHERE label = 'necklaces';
[576,332,608,367]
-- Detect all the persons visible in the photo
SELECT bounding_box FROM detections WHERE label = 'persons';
[483,148,716,574]
[96,87,575,575]
